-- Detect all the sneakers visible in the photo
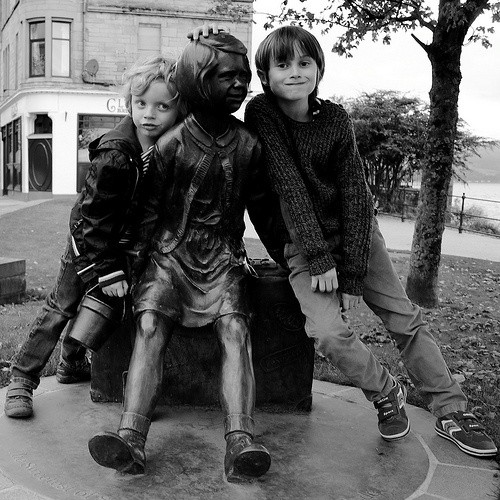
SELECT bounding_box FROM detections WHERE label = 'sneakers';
[433,410,497,456]
[373,375,410,441]
[5,382,33,418]
[56,356,91,383]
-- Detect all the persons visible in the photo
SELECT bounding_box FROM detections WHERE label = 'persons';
[87,29,272,481]
[3,25,228,419]
[245,26,498,458]
